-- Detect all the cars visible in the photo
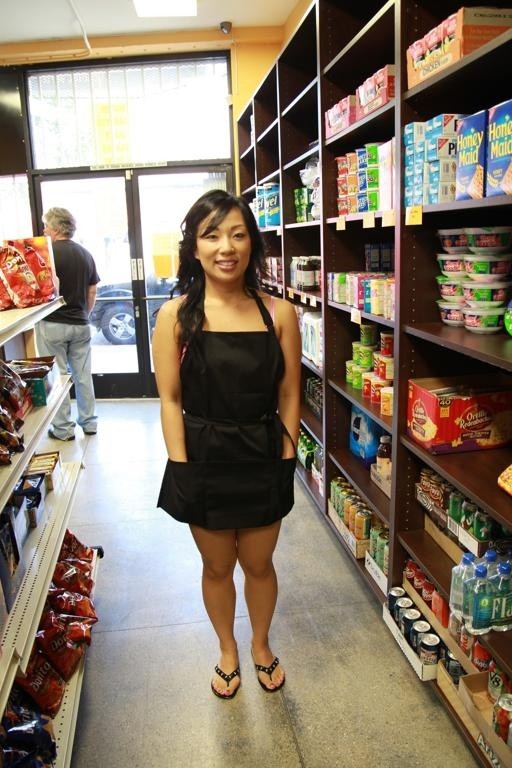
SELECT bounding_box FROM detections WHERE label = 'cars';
[89,277,192,345]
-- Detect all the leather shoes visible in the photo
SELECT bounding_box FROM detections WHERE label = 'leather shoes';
[48,426,76,440]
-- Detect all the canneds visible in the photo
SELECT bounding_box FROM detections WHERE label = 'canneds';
[390,557,512,748]
[419,467,503,543]
[329,476,389,579]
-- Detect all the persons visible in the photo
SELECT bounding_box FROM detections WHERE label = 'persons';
[32,205,105,444]
[148,189,305,700]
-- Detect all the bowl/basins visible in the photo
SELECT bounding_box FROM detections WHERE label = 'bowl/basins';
[434,225,512,335]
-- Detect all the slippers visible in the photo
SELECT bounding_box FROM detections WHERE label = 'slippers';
[211,665,240,699]
[255,656,285,692]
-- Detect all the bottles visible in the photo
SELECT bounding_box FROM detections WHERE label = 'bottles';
[377,436,392,480]
[298,428,324,482]
[288,255,321,291]
[447,547,511,637]
